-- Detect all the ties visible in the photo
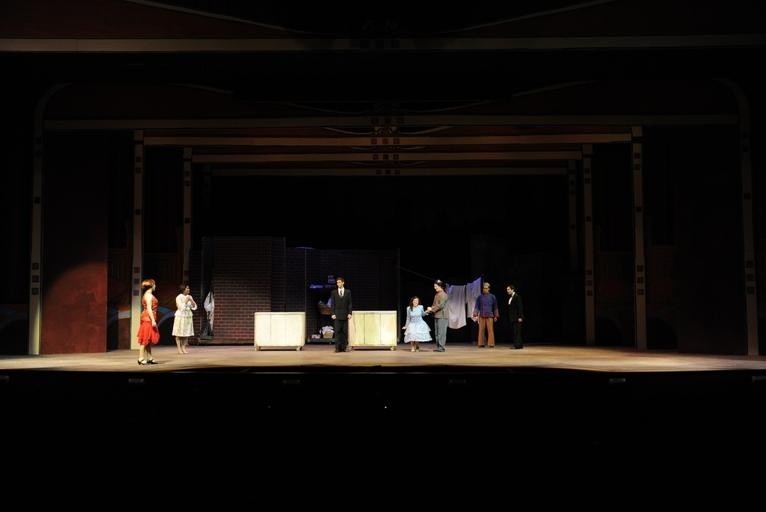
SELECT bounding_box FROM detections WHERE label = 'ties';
[339,289,343,296]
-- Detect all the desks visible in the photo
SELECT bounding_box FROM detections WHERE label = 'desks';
[252,310,308,352]
[347,308,400,351]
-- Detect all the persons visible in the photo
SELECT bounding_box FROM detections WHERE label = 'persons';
[473,282,499,348]
[330,277,352,352]
[401,296,433,352]
[137,279,159,365]
[171,284,197,354]
[427,280,449,352]
[506,285,524,349]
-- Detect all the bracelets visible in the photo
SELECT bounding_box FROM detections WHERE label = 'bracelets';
[152,320,156,322]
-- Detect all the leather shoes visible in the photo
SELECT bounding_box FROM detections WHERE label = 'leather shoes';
[137,359,159,366]
